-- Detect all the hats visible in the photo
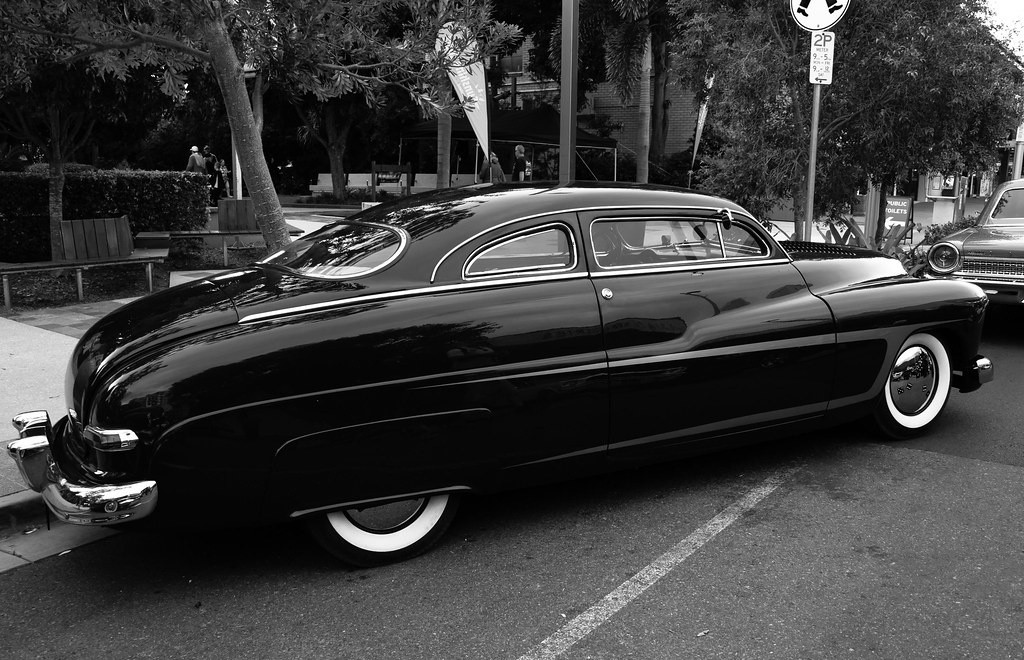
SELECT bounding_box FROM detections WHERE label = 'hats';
[190,146,198,151]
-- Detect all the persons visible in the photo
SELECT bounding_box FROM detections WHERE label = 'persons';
[479,152,506,185]
[186,145,234,206]
[512,145,530,181]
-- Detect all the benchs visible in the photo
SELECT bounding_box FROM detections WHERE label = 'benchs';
[0,197,304,309]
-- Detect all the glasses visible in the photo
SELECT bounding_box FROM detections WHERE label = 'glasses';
[205,148,211,150]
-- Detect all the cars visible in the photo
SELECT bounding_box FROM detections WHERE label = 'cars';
[6,186,995,563]
[925,176,1024,303]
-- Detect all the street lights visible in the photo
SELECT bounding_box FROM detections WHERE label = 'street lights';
[217,60,264,232]
